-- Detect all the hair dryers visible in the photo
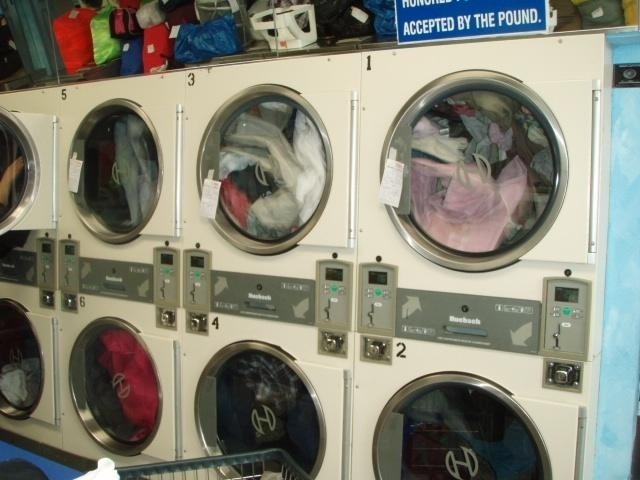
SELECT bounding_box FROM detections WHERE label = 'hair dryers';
[0,279,62,466]
[177,310,351,480]
[55,289,180,480]
[0,82,52,287]
[54,67,176,307]
[348,332,602,480]
[355,33,617,363]
[178,44,354,335]
